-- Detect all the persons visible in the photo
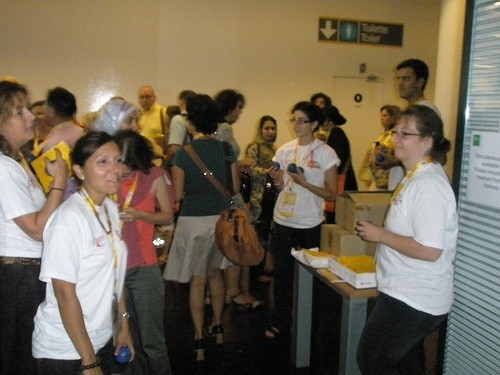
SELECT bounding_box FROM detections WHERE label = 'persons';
[244,92,359,339]
[0,76,259,375]
[355,58,460,375]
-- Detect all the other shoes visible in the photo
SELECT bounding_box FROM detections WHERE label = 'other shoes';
[260,270,272,283]
[226,291,260,310]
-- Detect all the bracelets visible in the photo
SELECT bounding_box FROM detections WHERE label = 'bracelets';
[50,187,64,191]
[76,354,102,371]
[119,312,129,318]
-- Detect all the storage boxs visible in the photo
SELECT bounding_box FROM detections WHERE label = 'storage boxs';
[319,191,395,289]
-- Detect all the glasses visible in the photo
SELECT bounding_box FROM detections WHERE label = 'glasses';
[390,128,423,139]
[289,118,311,125]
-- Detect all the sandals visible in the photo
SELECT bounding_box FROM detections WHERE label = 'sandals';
[265,324,285,338]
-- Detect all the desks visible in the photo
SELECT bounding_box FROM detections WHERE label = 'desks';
[288,258,439,375]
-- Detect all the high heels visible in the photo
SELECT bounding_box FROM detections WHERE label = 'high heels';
[191,338,206,360]
[206,323,224,345]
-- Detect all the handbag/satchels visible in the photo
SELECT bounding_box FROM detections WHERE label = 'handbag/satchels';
[215,205,265,266]
[324,174,345,212]
